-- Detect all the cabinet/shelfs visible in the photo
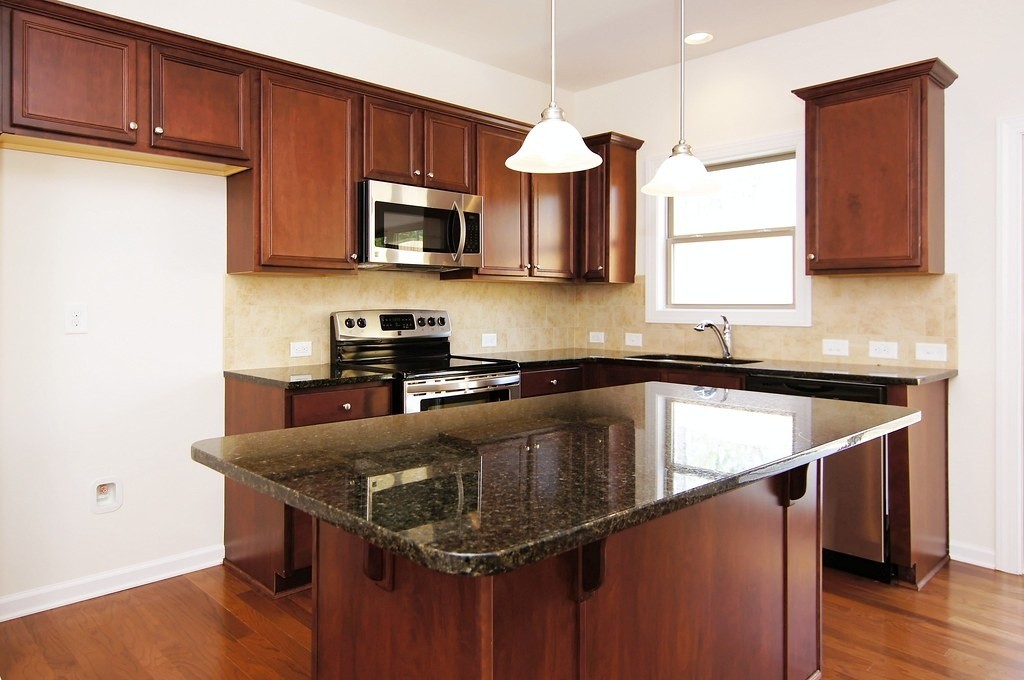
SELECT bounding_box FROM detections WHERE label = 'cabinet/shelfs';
[580,133,645,286]
[363,82,478,194]
[791,56,958,274]
[0,0,253,176]
[521,362,746,397]
[473,107,579,286]
[227,52,363,276]
[224,378,397,599]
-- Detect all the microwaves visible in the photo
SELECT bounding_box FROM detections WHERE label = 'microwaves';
[343,444,484,543]
[358,179,485,273]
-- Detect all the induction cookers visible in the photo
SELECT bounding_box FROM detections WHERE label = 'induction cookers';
[330,309,519,375]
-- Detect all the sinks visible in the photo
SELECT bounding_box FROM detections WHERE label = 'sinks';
[621,353,764,367]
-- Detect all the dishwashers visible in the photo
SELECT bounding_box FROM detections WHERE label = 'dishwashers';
[742,374,891,568]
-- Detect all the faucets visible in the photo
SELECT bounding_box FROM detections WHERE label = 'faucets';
[693,314,733,360]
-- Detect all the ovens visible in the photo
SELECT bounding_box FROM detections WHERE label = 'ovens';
[393,374,522,415]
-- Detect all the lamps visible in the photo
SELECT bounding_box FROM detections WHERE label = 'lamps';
[641,0,715,196]
[506,0,603,174]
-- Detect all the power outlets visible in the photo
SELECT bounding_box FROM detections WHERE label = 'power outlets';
[869,341,897,358]
[290,341,312,357]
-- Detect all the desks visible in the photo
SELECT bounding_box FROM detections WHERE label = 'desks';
[192,382,923,679]
